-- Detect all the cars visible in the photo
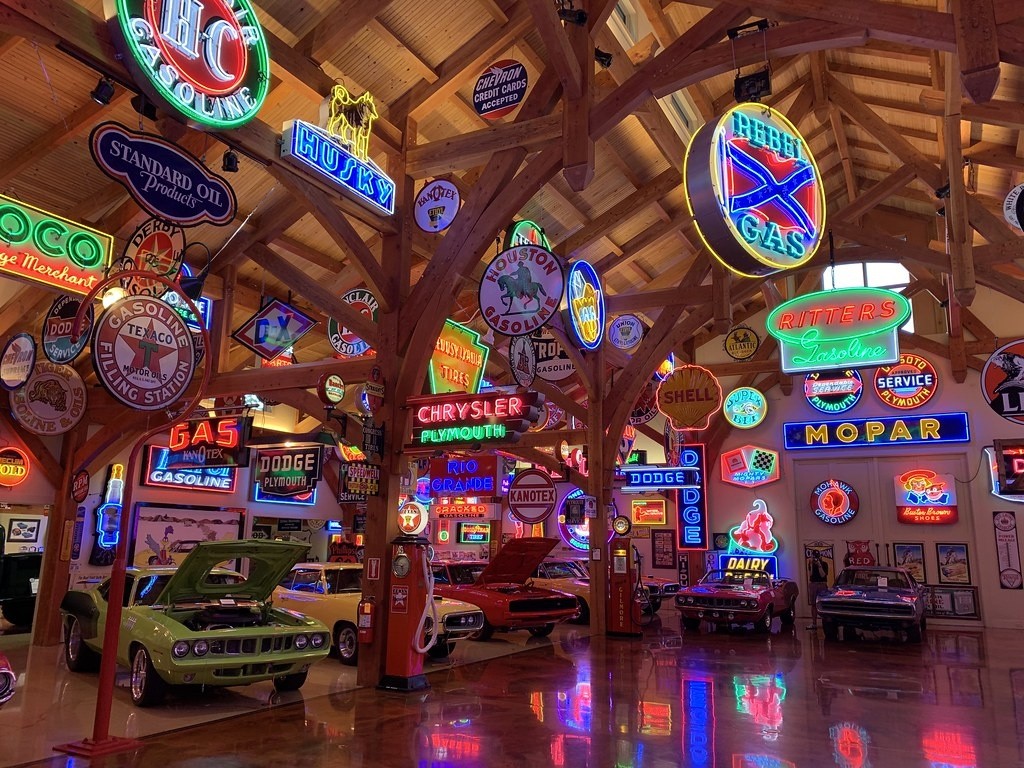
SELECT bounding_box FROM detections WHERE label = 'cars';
[429,538,580,643]
[562,557,680,613]
[673,568,799,635]
[815,565,927,644]
[523,557,649,626]
[61,539,332,707]
[265,562,486,667]
